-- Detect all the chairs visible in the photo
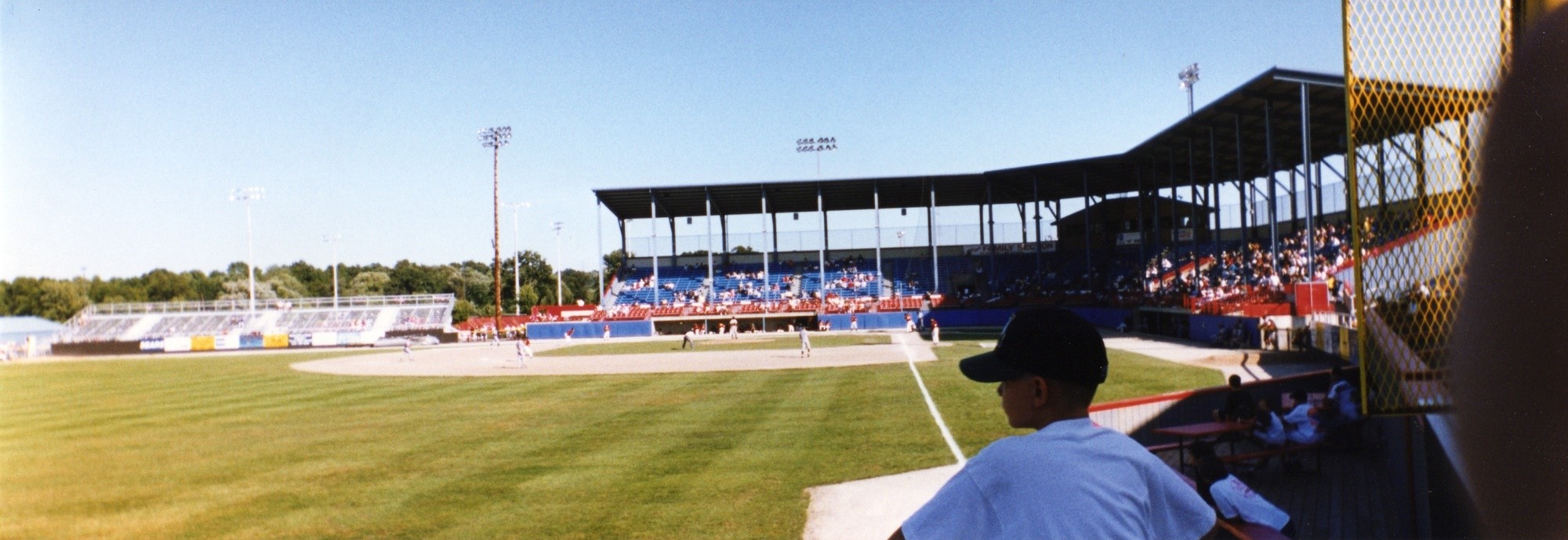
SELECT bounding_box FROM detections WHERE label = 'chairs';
[556,293,956,322]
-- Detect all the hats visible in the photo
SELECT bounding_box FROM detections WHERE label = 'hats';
[959,305,1108,383]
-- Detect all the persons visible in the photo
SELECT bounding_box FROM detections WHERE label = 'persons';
[956,216,1378,350]
[400,337,412,358]
[564,328,574,341]
[1453,0,1568,540]
[517,334,533,370]
[799,326,810,357]
[1188,441,1297,540]
[596,254,939,343]
[683,330,694,350]
[889,306,1219,540]
[474,313,558,346]
[1225,366,1371,444]
[603,324,610,340]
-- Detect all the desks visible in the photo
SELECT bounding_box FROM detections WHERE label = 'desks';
[1153,417,1286,489]
[1282,393,1329,413]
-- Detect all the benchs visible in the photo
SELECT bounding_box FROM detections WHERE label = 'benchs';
[615,254,957,304]
[1145,441,1342,469]
